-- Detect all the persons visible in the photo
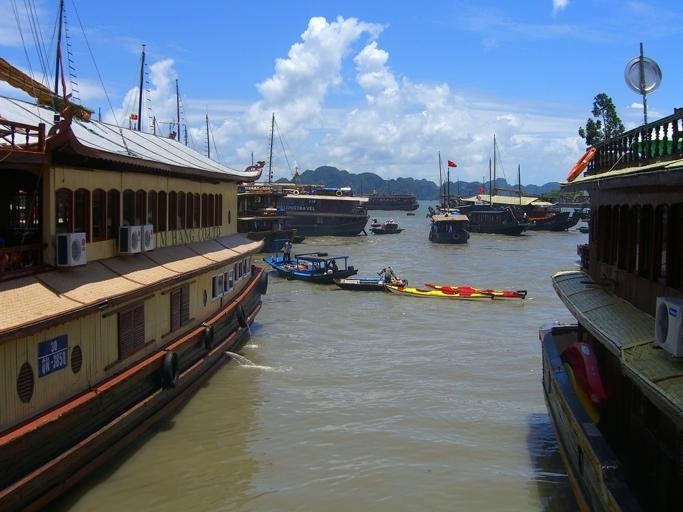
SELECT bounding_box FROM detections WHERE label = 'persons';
[379,266,399,283]
[282,238,292,261]
[328,260,338,275]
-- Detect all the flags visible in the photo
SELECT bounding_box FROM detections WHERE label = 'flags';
[447,160,456,167]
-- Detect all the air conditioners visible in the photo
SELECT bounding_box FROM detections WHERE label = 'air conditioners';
[120,224,154,255]
[56,232,87,268]
[654,295,683,358]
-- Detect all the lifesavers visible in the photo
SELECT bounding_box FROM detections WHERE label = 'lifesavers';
[293,190,299,196]
[336,191,342,196]
[566,148,597,182]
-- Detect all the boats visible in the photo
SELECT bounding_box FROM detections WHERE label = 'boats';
[353,187,419,210]
[0,0,266,512]
[429,132,583,244]
[335,276,408,291]
[237,111,371,252]
[386,284,527,301]
[538,42,682,512]
[263,252,358,284]
[369,218,406,234]
